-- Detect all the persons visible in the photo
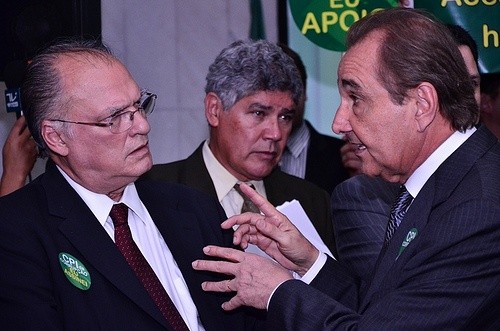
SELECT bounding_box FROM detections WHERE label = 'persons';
[271,45,362,196]
[0,115,39,197]
[0,40,264,331]
[333,26,480,281]
[191,9,500,331]
[477,72,500,141]
[138,39,335,250]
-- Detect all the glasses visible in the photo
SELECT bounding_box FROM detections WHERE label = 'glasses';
[46,90,158,134]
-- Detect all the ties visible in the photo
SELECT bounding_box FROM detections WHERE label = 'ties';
[233,184,265,214]
[382,187,414,244]
[109,203,191,331]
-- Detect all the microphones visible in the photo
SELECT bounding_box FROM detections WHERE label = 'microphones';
[4,71,23,120]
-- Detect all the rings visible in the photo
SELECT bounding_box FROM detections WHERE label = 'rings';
[227,279,233,291]
